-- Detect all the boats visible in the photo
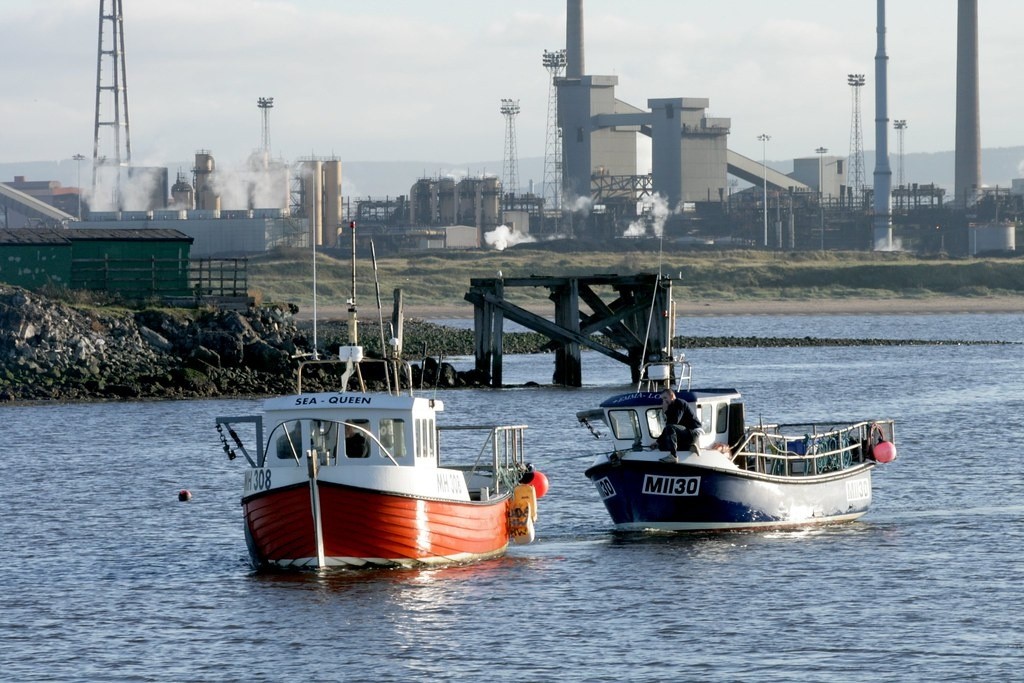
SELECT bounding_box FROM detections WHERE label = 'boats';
[575,277,897,533]
[216,169,551,574]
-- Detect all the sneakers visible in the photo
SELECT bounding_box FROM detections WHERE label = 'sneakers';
[689,444,700,456]
[659,454,678,463]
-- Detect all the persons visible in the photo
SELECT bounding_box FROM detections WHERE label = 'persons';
[277,420,302,458]
[332,419,371,458]
[650,389,701,463]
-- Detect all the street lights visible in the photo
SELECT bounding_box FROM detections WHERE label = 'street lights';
[757,133,771,247]
[815,145,828,249]
[73,154,86,219]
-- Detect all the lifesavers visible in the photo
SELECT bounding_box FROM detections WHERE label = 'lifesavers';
[713,442,732,460]
[868,423,884,462]
[509,499,536,544]
